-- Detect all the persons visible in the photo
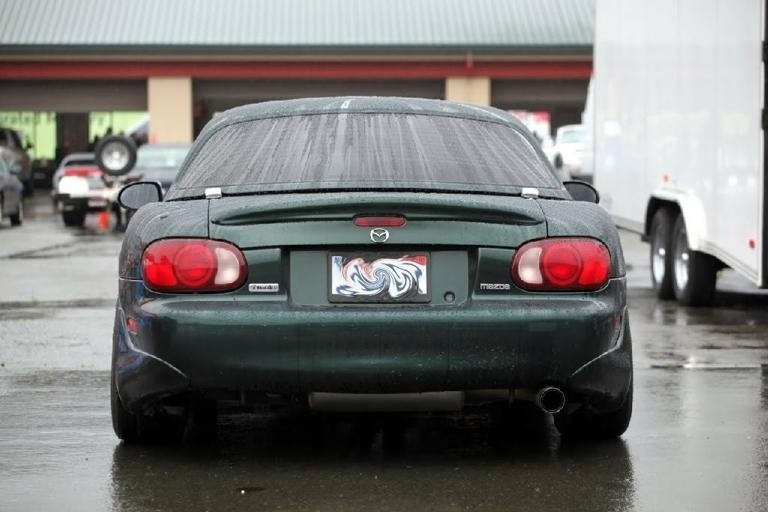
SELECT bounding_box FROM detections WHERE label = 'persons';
[87,127,148,151]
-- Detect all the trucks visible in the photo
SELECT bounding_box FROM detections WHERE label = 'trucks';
[583,0,766,302]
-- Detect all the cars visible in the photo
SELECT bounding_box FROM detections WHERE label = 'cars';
[553,115,590,177]
[53,144,193,228]
[0,129,32,224]
[113,96,634,445]
